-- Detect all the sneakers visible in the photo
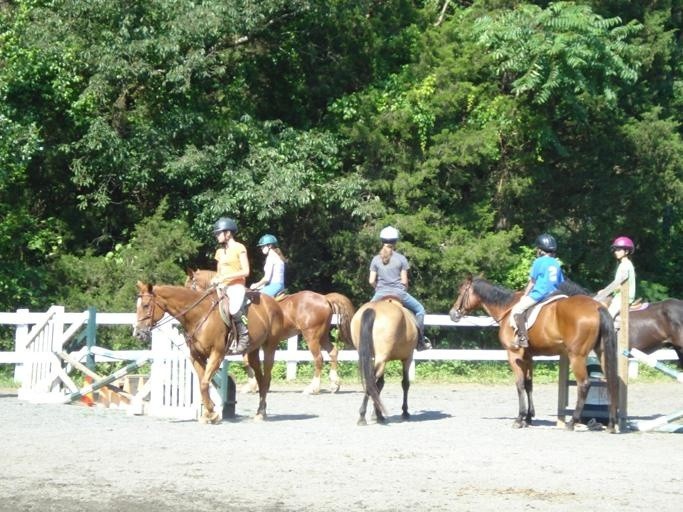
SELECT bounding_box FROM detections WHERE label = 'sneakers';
[511,337,528,347]
[417,341,431,351]
[232,335,250,355]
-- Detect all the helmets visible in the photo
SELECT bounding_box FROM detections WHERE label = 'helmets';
[380,226,398,239]
[535,233,556,251]
[609,237,635,254]
[257,233,276,247]
[211,218,239,234]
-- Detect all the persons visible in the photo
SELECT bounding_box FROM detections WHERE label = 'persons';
[508,232,567,349]
[367,225,433,352]
[206,216,252,353]
[594,235,638,321]
[248,233,287,300]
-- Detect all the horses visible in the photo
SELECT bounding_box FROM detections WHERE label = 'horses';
[448,269,622,435]
[550,271,683,434]
[181,265,355,397]
[349,299,421,427]
[133,278,286,425]
